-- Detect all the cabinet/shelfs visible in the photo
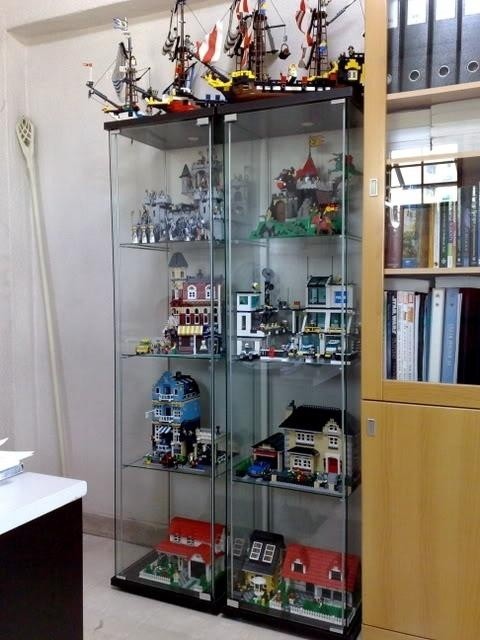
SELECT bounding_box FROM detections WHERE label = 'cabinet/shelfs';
[360,0,479,639]
[104,119,226,602]
[225,102,358,637]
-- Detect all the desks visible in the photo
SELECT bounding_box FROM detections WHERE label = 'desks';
[0,471,89,640]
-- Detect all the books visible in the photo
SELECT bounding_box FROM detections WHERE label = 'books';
[385,184,480,269]
[383,274,480,385]
